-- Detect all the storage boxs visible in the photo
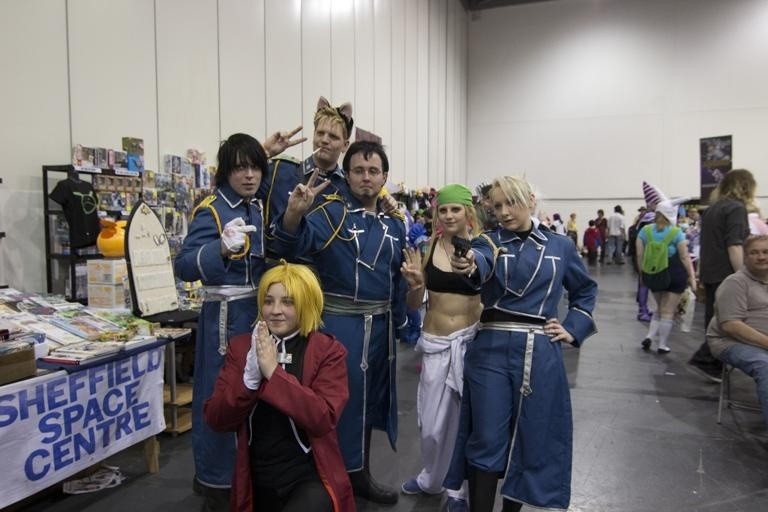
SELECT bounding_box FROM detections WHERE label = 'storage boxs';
[0,342,39,389]
[85,256,131,286]
[87,283,133,311]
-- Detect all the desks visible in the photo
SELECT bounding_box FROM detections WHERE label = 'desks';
[0,327,193,511]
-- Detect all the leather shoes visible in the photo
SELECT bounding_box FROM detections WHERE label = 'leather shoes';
[641,337,652,351]
[657,345,672,353]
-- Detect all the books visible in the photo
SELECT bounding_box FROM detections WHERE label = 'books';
[0,287,192,366]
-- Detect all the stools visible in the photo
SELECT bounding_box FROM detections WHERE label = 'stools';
[715,361,763,426]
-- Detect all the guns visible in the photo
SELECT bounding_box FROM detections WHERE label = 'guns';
[450,236,472,270]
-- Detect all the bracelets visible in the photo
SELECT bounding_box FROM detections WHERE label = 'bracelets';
[405,281,425,298]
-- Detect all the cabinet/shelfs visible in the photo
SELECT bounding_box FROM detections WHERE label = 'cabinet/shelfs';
[140,308,201,439]
[42,163,142,306]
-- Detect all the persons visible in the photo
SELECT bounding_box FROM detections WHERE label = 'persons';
[451,174,599,511]
[399,184,485,509]
[537,204,761,355]
[704,234,768,428]
[173,132,268,511]
[47,172,101,249]
[254,95,399,266]
[264,139,408,506]
[202,257,358,511]
[685,168,758,384]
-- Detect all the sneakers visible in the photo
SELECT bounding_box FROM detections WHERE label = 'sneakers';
[400,474,445,497]
[192,472,233,511]
[686,356,725,384]
[446,493,470,512]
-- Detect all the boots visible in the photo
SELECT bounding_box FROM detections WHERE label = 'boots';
[636,283,653,323]
[468,473,499,512]
[502,497,523,512]
[346,422,400,506]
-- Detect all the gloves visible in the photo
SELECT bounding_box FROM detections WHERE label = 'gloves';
[242,320,268,392]
[219,216,257,254]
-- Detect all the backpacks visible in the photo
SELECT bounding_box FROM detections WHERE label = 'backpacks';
[641,224,682,294]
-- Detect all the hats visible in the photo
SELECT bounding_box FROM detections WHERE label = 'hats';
[657,201,677,224]
